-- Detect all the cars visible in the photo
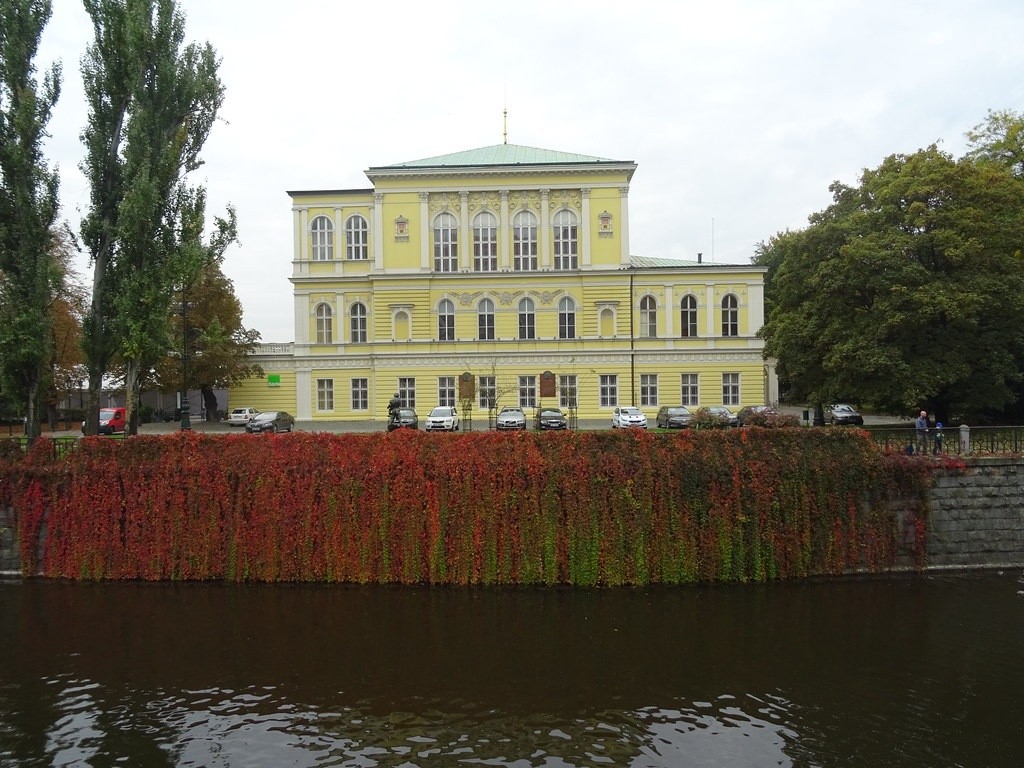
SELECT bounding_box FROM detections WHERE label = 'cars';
[737,405,778,428]
[611,406,648,429]
[535,408,567,430]
[823,404,863,426]
[696,405,740,428]
[245,410,295,435]
[387,407,419,432]
[425,406,460,433]
[81,407,126,436]
[656,405,694,429]
[228,407,261,427]
[495,405,527,432]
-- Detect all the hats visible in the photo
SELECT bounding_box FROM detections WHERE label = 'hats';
[936,422,941,427]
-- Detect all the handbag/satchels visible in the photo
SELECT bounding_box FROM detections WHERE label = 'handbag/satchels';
[905,444,914,456]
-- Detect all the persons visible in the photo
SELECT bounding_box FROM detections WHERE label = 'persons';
[932,423,944,451]
[916,411,929,454]
[387,392,401,427]
